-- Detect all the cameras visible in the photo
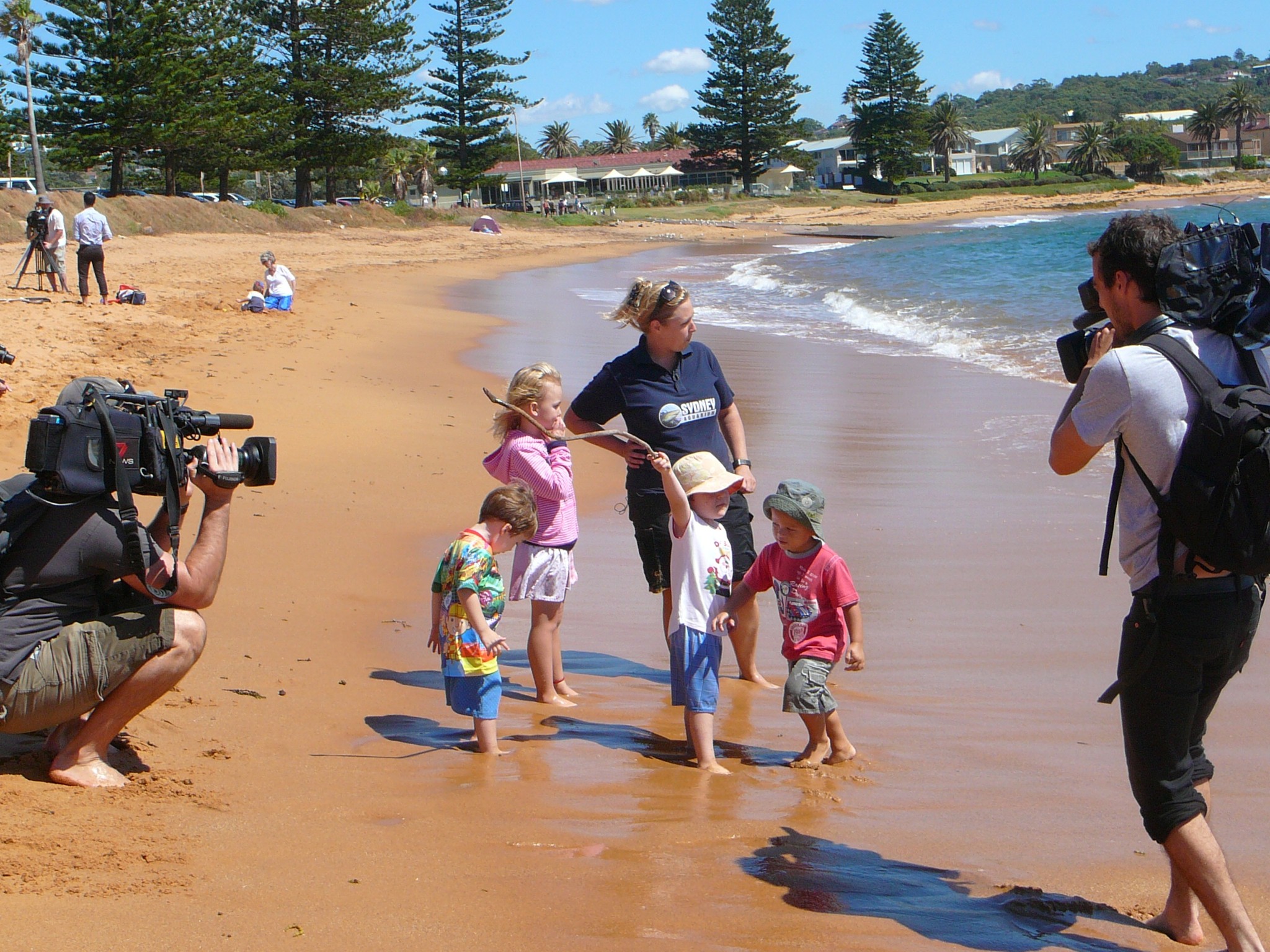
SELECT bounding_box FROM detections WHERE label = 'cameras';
[1,345,15,365]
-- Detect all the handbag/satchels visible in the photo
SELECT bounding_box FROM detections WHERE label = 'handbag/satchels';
[116,288,147,305]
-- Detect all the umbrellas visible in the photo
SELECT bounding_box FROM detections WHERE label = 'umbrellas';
[657,166,685,190]
[780,164,805,174]
[629,167,657,194]
[601,169,629,196]
[541,171,587,201]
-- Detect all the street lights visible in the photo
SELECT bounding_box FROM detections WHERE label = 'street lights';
[480,98,527,214]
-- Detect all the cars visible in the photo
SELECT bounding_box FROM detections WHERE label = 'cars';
[0,177,396,210]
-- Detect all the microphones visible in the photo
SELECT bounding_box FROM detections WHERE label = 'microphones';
[188,413,253,431]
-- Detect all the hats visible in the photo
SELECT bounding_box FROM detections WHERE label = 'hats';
[35,195,54,204]
[762,478,827,545]
[255,280,263,288]
[672,451,745,498]
[261,251,275,263]
[56,374,155,411]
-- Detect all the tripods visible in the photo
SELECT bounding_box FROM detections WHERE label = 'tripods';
[15,238,70,294]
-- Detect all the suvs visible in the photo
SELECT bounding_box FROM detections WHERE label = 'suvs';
[484,200,534,213]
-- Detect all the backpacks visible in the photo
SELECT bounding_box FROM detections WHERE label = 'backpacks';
[0,474,99,616]
[1113,334,1269,581]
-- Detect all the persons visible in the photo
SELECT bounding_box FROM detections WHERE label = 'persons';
[709,480,866,771]
[0,374,242,792]
[561,275,781,691]
[481,224,494,234]
[236,280,267,313]
[34,194,71,294]
[423,478,541,755]
[537,185,740,218]
[1044,209,1270,952]
[646,451,736,776]
[463,190,471,208]
[479,363,584,709]
[431,190,438,208]
[473,197,479,209]
[259,249,298,312]
[422,192,430,208]
[72,190,113,306]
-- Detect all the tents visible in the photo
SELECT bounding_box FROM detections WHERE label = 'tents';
[469,215,502,236]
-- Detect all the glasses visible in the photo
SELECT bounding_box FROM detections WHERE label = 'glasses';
[649,280,680,320]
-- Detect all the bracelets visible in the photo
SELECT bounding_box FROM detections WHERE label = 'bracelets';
[160,496,190,515]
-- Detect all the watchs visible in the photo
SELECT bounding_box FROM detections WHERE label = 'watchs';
[730,459,752,469]
